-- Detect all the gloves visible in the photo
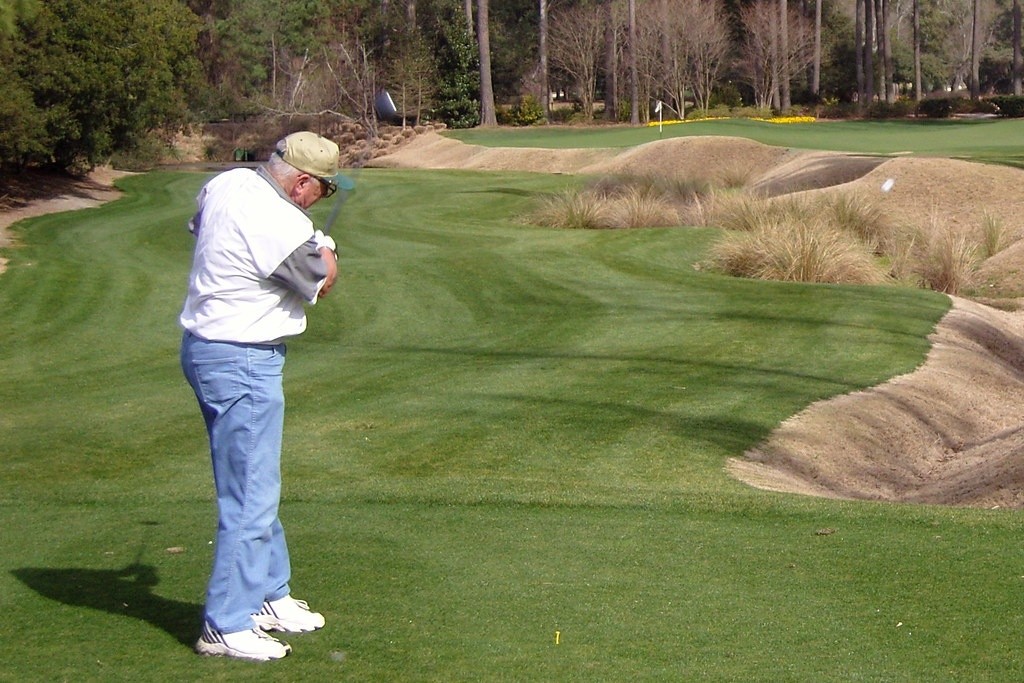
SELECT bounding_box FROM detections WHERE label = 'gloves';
[314,229,339,261]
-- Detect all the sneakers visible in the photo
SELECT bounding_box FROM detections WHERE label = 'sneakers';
[250,593,326,633]
[195,621,293,660]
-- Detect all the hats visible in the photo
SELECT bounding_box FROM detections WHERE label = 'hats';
[275,131,355,190]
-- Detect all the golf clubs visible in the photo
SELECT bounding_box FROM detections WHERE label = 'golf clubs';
[321,91,398,238]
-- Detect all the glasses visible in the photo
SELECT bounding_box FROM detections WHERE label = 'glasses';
[297,173,337,199]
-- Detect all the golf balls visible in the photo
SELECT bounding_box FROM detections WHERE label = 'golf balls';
[882,178,894,191]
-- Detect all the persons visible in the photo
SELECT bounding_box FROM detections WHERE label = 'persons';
[176,130,355,659]
